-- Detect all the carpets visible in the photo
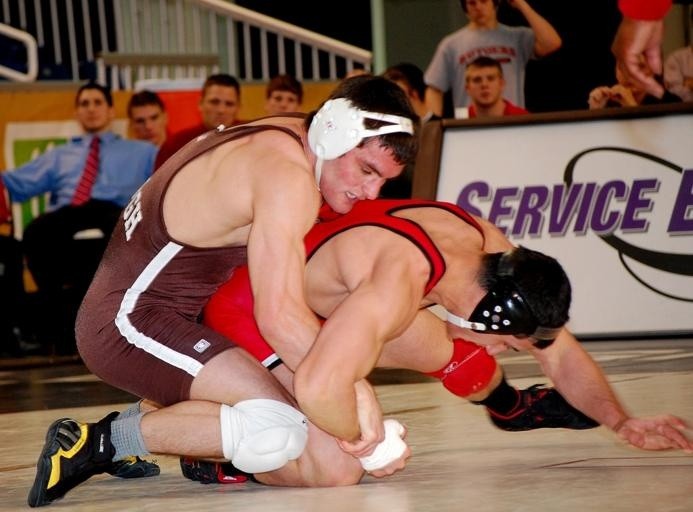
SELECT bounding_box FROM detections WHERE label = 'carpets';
[70,137,100,207]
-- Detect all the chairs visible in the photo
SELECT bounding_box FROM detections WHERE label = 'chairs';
[487,384,601,432]
[95,410,160,479]
[28,417,115,508]
[180,455,249,484]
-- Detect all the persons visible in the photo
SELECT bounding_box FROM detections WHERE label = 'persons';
[380,63,440,199]
[420,1,561,120]
[589,49,678,104]
[29,73,423,506]
[262,74,303,117]
[454,55,529,120]
[665,40,691,109]
[1,81,161,352]
[0,182,23,354]
[153,73,250,175]
[127,88,168,148]
[610,2,672,99]
[180,198,693,487]
